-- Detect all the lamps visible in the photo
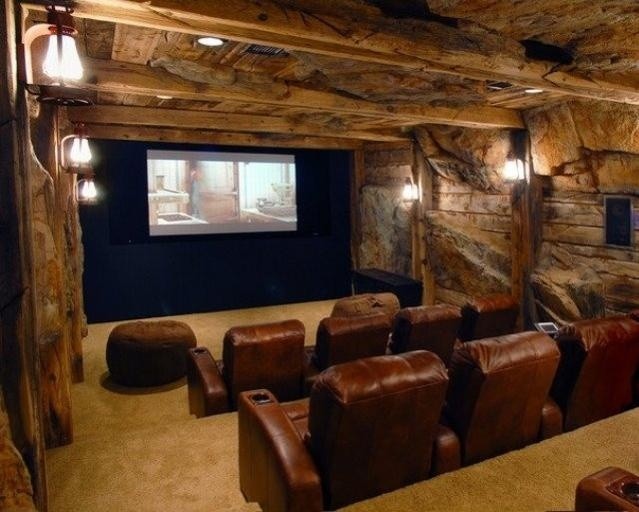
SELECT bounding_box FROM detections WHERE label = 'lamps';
[501,151,527,184]
[401,177,421,205]
[22,22,104,206]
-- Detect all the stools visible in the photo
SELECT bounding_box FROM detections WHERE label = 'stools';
[352,267,423,309]
[104,319,197,389]
